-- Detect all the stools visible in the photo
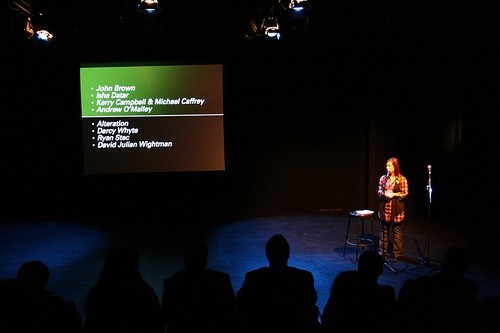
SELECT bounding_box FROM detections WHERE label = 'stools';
[342,209,376,265]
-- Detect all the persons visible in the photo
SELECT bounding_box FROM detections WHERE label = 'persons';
[0,261,84,333]
[397,242,481,333]
[236,234,321,333]
[377,158,409,258]
[86,243,162,333]
[162,240,236,333]
[321,250,397,333]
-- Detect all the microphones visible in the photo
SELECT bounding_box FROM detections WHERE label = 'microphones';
[427,165,432,174]
